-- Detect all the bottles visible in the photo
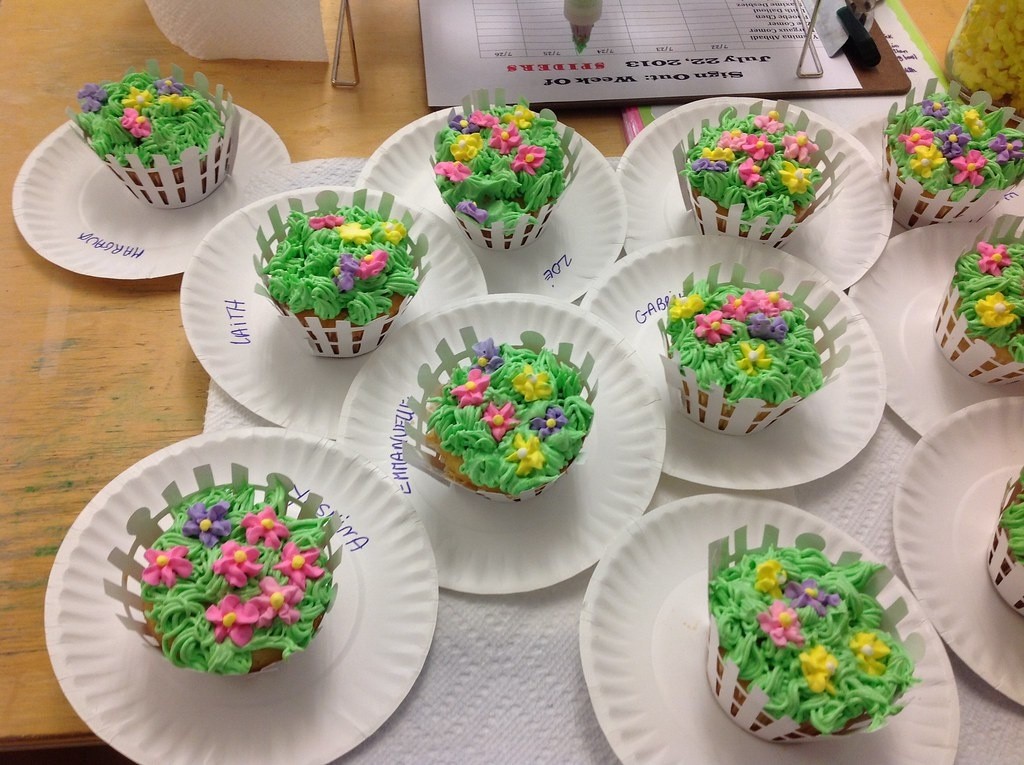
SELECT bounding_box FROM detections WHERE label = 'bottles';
[943,0,1024,129]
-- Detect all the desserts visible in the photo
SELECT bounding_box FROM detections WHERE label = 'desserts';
[989,470,1024,610]
[422,335,596,495]
[77,71,229,204]
[678,109,823,253]
[881,91,1024,227]
[706,543,923,743]
[937,241,1024,386]
[137,482,332,678]
[434,105,567,250]
[664,279,825,436]
[262,204,420,356]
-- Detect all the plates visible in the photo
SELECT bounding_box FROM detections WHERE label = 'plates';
[891,396,1024,706]
[848,222,1023,438]
[852,119,1023,237]
[12,99,290,280]
[334,293,667,595]
[180,185,489,440]
[354,106,626,302]
[578,494,959,764]
[44,428,439,765]
[578,235,887,490]
[615,97,893,291]
